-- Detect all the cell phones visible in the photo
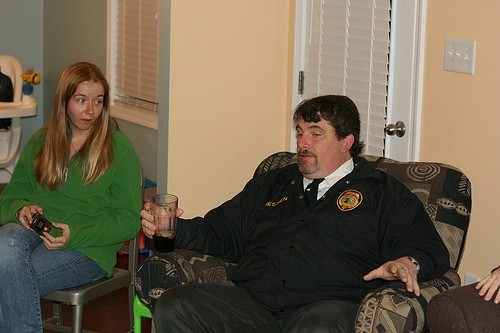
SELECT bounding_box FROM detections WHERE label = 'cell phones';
[30,214,52,235]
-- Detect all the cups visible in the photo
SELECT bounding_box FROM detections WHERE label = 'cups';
[150,192,178,252]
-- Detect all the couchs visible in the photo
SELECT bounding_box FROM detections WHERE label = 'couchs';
[135,151,472,333]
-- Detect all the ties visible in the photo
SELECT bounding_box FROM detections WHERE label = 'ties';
[304,178,325,207]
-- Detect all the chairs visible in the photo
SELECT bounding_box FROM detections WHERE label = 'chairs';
[0,54,38,183]
[40,162,144,333]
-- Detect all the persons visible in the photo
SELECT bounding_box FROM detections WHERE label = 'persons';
[0,62,142,333]
[141,95,450,333]
[476,268,500,304]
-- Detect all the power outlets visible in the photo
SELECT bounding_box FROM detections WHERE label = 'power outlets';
[464,273,480,286]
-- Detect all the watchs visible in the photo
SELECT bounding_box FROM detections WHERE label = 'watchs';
[402,256,420,273]
